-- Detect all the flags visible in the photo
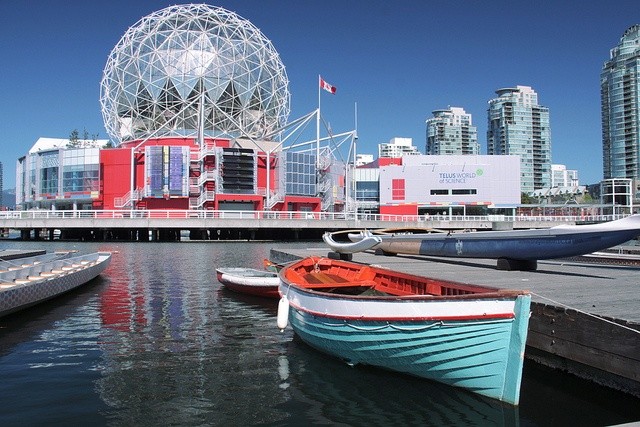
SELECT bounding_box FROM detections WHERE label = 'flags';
[320,78,337,95]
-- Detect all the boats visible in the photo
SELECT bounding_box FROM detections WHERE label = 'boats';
[277,254,532,406]
[347,212,640,259]
[0,251,112,316]
[216,266,280,298]
[0,251,72,274]
